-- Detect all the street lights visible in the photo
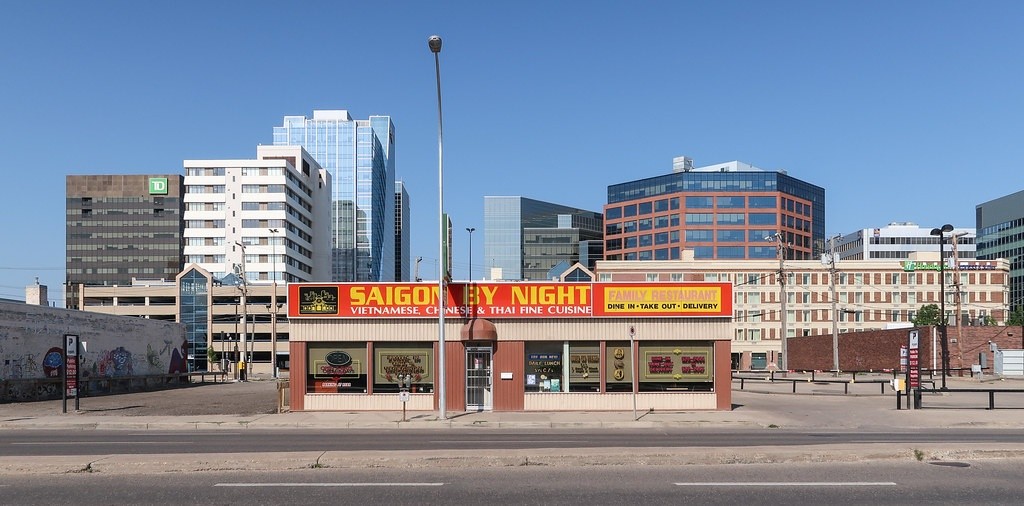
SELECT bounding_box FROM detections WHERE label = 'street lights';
[427,35,446,419]
[930,224,953,391]
[466,228,475,282]
